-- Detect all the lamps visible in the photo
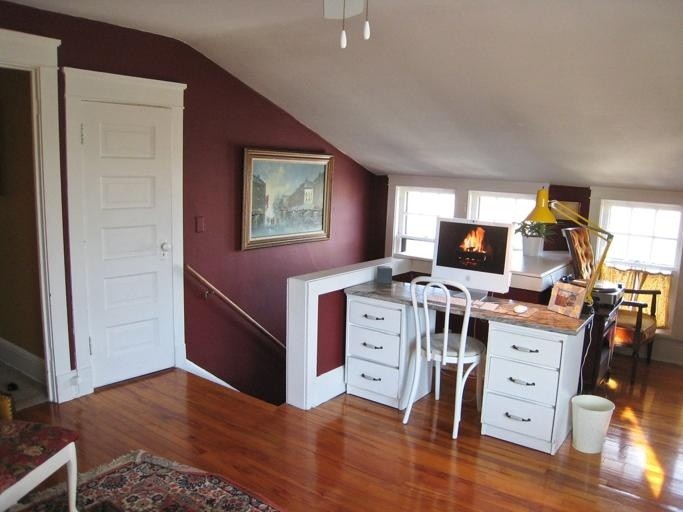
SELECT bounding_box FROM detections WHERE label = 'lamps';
[523,185,614,307]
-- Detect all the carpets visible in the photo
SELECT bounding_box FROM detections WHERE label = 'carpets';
[0,448,278,512]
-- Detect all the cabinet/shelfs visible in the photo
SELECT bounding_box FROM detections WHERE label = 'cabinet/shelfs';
[343,273,595,457]
[582,297,624,395]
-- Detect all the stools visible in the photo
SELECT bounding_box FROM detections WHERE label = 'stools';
[1,416,79,512]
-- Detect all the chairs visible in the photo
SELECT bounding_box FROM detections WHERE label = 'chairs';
[560,225,661,387]
[402,276,487,440]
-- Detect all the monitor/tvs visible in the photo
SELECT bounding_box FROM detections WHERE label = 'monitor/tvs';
[430,217,514,301]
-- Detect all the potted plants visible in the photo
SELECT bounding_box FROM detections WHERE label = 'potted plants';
[516,222,557,258]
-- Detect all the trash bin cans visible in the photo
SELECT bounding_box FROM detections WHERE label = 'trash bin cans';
[571,394,616,454]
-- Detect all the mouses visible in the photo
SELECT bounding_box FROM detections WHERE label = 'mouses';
[512,304,527,314]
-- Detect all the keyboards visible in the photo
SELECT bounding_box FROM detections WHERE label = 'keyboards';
[426,294,498,311]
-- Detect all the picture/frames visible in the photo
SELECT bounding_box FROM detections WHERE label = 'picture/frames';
[547,282,588,321]
[239,145,336,252]
